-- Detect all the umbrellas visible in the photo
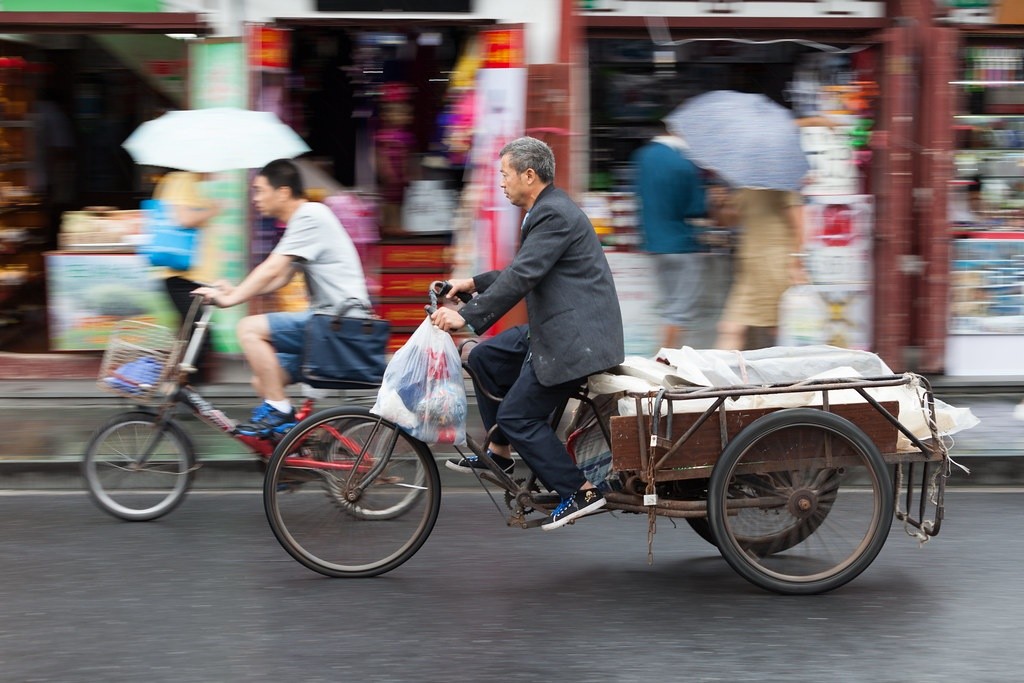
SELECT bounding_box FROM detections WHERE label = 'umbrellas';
[663,92,809,194]
[122,109,310,175]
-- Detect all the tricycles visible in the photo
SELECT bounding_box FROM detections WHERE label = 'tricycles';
[262,281,948,594]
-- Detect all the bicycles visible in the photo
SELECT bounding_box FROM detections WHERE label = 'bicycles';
[82,286,431,522]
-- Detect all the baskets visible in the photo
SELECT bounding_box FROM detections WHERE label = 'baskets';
[97,320,183,404]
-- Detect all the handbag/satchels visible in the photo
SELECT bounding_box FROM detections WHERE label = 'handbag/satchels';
[368,314,467,448]
[777,282,828,346]
[303,297,391,383]
[138,200,198,271]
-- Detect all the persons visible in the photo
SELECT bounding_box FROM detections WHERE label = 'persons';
[152,171,223,386]
[430,138,625,530]
[191,158,370,494]
[636,117,808,352]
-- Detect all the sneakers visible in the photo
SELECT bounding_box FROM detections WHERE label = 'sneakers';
[235,402,298,436]
[541,488,607,531]
[445,448,515,478]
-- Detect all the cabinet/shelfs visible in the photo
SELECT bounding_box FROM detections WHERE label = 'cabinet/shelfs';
[0,48,71,346]
[942,37,1024,373]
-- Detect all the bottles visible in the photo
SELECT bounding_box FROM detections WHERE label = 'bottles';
[412,425,465,444]
